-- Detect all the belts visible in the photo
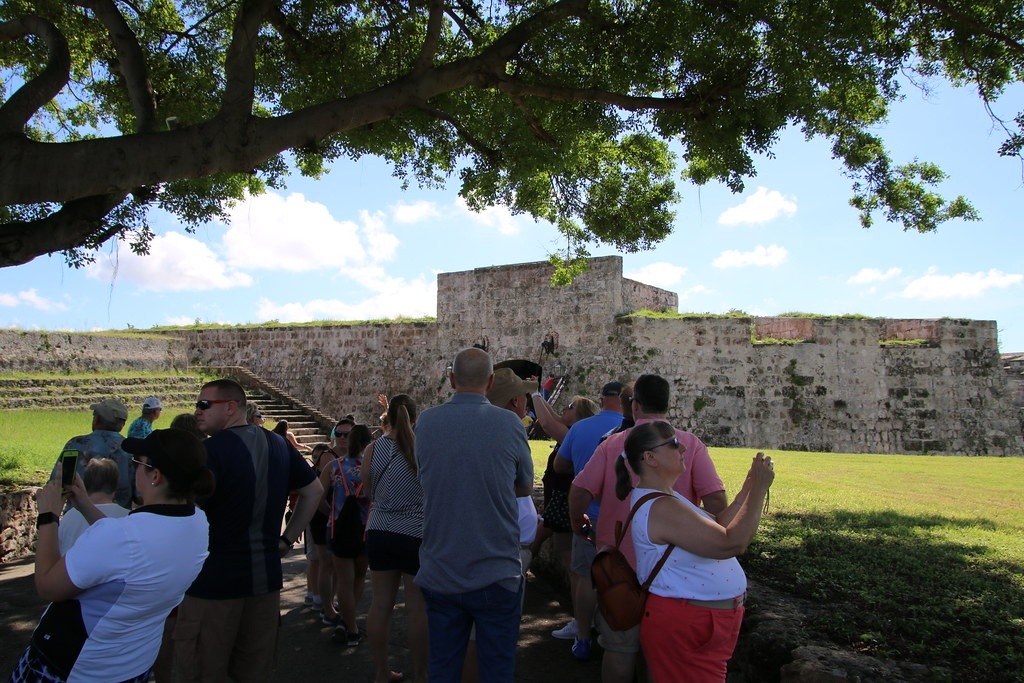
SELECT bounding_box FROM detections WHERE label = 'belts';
[686,594,748,610]
[519,544,530,549]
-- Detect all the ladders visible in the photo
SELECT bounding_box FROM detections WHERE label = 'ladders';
[528,369,571,441]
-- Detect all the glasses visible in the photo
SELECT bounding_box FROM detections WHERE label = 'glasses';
[313,455,317,459]
[335,431,349,438]
[255,414,261,419]
[195,400,239,411]
[642,437,678,461]
[568,401,577,410]
[131,456,152,470]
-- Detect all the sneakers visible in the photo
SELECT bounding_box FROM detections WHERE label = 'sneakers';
[332,623,347,643]
[319,606,342,625]
[572,635,592,660]
[551,618,578,640]
[347,628,361,644]
[304,596,313,605]
[312,602,339,612]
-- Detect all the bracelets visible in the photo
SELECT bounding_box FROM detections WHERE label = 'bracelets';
[280,535,292,547]
[531,392,541,398]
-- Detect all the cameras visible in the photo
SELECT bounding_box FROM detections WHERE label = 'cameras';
[761,458,774,470]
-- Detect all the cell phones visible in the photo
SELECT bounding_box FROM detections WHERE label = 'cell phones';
[60,449,80,494]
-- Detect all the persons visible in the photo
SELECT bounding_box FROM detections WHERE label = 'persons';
[35,426,209,683]
[313,365,639,683]
[414,347,534,683]
[614,422,774,683]
[567,374,728,683]
[50,379,323,683]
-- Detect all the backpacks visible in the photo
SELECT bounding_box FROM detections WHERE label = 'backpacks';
[592,491,677,633]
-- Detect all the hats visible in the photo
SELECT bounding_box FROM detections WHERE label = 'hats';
[90,400,127,423]
[602,381,625,395]
[485,367,538,409]
[142,398,161,410]
[121,428,207,470]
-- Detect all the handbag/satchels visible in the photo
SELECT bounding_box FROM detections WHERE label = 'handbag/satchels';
[542,488,573,528]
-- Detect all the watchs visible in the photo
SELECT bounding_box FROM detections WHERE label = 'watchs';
[37,512,60,529]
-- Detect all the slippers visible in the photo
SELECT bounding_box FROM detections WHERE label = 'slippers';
[373,670,403,683]
